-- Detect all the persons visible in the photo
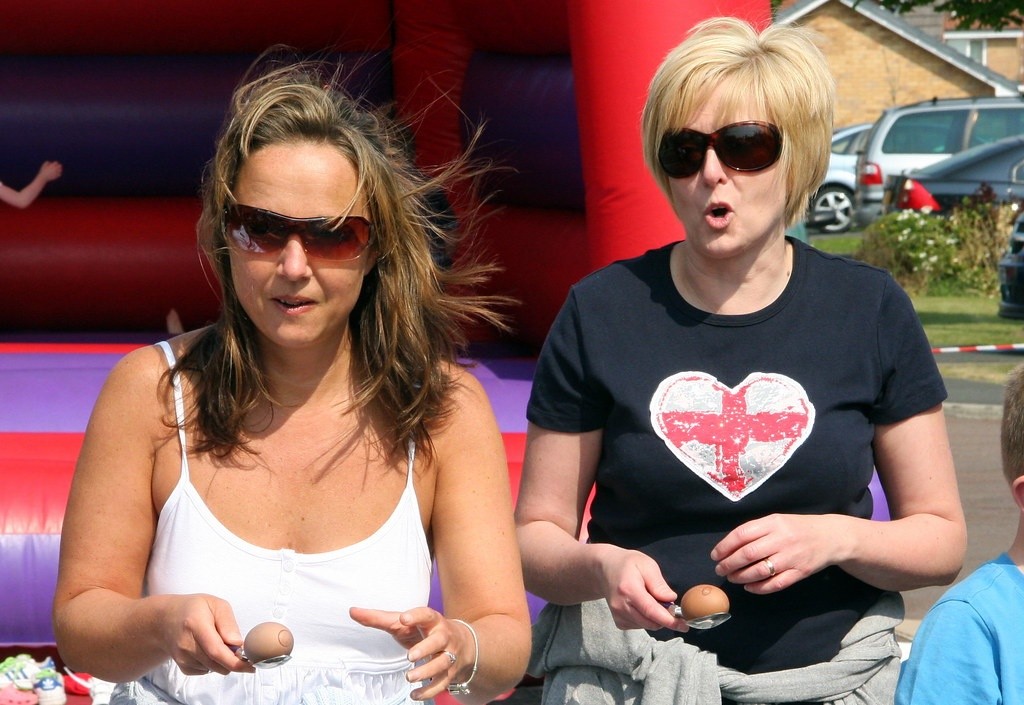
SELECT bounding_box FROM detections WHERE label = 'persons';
[894,361,1023,705]
[515,16,966,704]
[0,161,61,210]
[51,65,532,705]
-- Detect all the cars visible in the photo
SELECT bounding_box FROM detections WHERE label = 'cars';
[881,135,1024,216]
[806,124,990,234]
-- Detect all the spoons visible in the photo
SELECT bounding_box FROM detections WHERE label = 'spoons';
[229,645,293,669]
[660,602,732,630]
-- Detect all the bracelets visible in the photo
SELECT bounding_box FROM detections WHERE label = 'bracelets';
[445,619,478,695]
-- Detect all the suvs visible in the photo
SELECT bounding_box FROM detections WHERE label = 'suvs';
[853,96,1024,225]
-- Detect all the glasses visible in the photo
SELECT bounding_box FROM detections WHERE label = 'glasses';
[221,203,374,260]
[657,121,783,178]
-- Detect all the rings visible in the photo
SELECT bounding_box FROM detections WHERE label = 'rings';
[444,651,456,663]
[765,558,775,576]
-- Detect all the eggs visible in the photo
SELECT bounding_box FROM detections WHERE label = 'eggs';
[243,621,293,663]
[681,584,729,622]
